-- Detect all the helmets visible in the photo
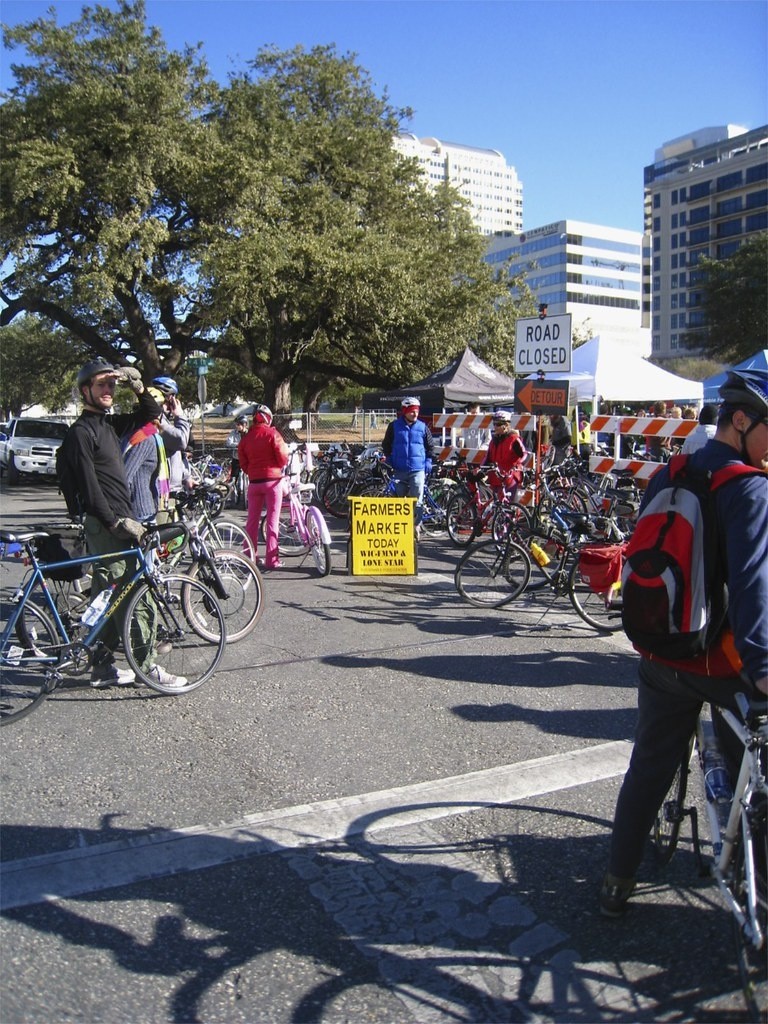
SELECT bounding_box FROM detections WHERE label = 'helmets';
[147,387,165,405]
[401,397,421,406]
[78,359,114,388]
[152,378,178,396]
[253,404,272,425]
[492,410,511,422]
[234,415,249,427]
[719,350,768,411]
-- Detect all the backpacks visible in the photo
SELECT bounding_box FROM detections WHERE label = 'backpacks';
[55,420,101,516]
[622,454,768,679]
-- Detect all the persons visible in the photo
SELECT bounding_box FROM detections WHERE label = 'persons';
[238,404,291,570]
[62,356,203,694]
[225,415,250,509]
[596,346,768,919]
[381,397,435,544]
[369,410,379,430]
[447,389,730,537]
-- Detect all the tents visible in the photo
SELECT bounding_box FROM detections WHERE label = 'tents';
[361,347,517,446]
[669,349,768,406]
[519,333,705,457]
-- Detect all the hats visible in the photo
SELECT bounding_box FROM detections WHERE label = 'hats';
[699,405,719,424]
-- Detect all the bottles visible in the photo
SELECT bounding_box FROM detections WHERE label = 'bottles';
[702,736,733,806]
[167,533,184,552]
[530,541,550,566]
[82,583,117,626]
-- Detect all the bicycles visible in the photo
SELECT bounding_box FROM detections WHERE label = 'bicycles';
[306,441,768,1024]
[261,445,332,577]
[15,479,267,659]
[189,450,331,512]
[0,526,227,727]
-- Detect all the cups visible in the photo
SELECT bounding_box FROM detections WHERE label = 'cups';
[458,438,465,448]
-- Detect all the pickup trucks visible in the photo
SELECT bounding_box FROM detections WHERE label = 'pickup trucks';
[0,417,71,483]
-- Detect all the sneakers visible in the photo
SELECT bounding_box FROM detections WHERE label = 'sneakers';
[600,874,637,916]
[135,665,188,689]
[90,666,135,687]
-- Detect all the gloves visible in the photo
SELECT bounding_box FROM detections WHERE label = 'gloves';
[425,459,433,473]
[113,519,151,547]
[116,364,143,393]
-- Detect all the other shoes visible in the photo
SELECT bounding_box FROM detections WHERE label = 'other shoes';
[267,561,285,568]
[156,640,172,654]
[257,558,265,566]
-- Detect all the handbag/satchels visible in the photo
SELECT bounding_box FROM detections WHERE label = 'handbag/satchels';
[580,544,622,593]
[35,525,91,581]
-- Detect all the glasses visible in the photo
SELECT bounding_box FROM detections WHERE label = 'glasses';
[493,422,504,426]
